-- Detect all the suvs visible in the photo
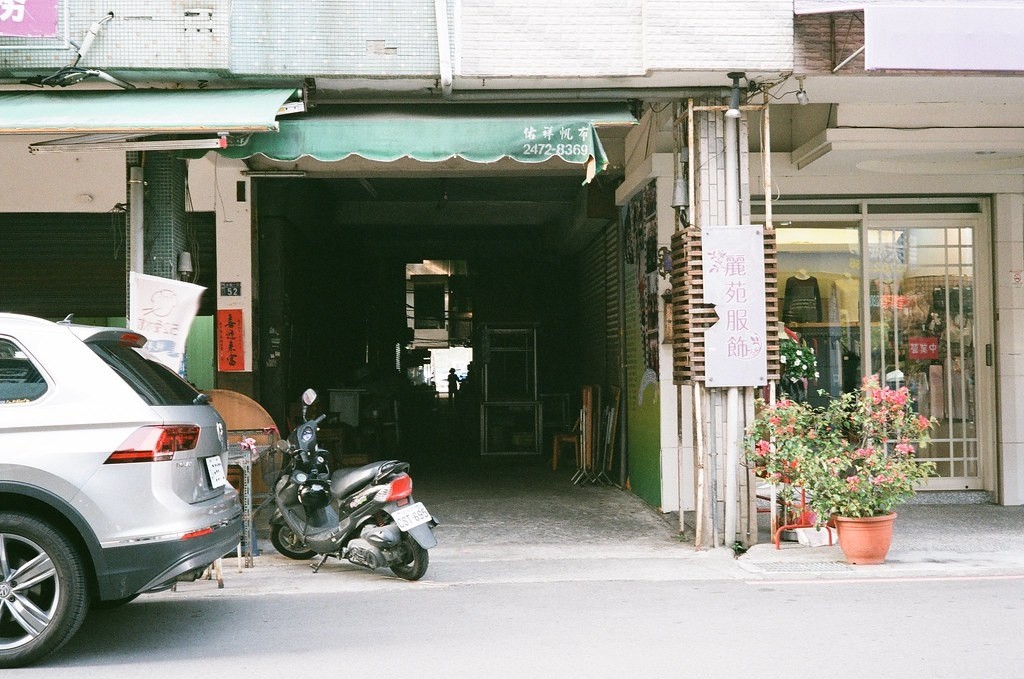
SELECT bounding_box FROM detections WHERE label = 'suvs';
[0,310,245,670]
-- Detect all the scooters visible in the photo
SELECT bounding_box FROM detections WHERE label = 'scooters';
[267,387,439,582]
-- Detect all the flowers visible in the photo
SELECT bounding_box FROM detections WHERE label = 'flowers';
[734,341,941,532]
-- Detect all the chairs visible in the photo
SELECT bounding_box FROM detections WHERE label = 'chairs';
[552,416,581,471]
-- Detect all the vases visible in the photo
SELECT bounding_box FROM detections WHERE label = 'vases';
[829,511,897,564]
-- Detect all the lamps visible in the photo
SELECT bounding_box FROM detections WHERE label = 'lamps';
[725,72,747,119]
[795,76,809,106]
[672,179,689,212]
[178,252,193,277]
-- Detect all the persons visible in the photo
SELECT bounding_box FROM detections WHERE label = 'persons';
[782,268,902,325]
[446,368,460,406]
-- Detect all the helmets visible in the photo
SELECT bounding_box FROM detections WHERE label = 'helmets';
[299,480,332,508]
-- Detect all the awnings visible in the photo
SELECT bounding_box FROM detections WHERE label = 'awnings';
[1,90,295,134]
[150,104,640,187]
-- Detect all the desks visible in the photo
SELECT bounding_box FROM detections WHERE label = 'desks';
[326,387,373,451]
[570,384,621,487]
